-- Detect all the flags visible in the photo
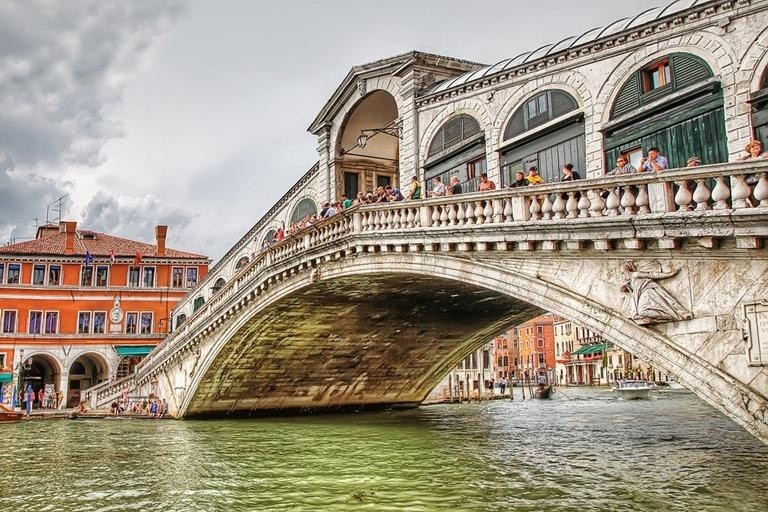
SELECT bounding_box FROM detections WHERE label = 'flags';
[110,248,115,267]
[132,251,143,269]
[84,250,93,265]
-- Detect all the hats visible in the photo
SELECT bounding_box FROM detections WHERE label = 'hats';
[685,156,702,164]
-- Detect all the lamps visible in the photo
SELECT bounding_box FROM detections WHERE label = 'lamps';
[357,126,403,149]
[158,317,172,329]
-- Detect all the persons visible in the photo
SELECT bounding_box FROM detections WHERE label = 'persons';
[523,166,546,218]
[404,176,422,201]
[605,155,638,198]
[233,185,404,279]
[445,176,464,195]
[506,171,533,188]
[619,259,693,325]
[735,140,768,207]
[537,372,546,391]
[686,156,702,209]
[475,172,496,208]
[488,377,494,394]
[19,386,64,414]
[500,377,506,394]
[558,163,582,215]
[638,146,670,173]
[108,393,169,419]
[429,177,444,198]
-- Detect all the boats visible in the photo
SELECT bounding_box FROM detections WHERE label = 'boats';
[535,385,554,399]
[0,404,23,421]
[615,380,650,400]
